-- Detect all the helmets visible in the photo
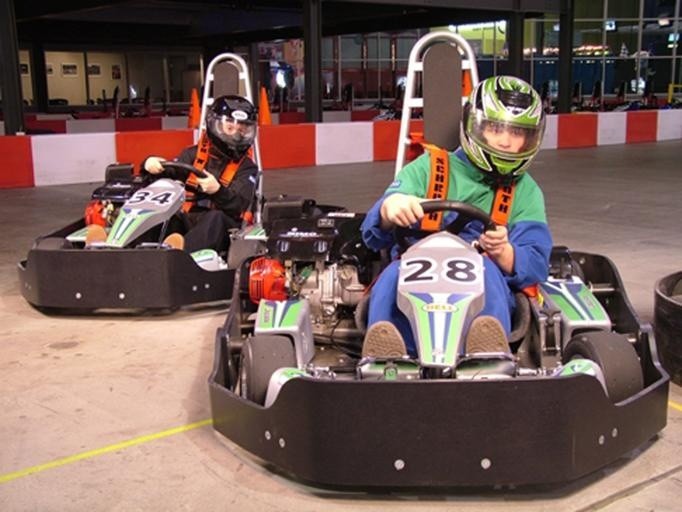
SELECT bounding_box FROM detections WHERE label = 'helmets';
[459,75,546,185]
[204,94,258,164]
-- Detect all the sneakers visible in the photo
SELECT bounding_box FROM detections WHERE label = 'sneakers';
[361,320,408,359]
[85,225,107,244]
[161,232,185,251]
[465,315,511,352]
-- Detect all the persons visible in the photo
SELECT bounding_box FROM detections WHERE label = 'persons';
[85,94,260,255]
[357,75,555,370]
